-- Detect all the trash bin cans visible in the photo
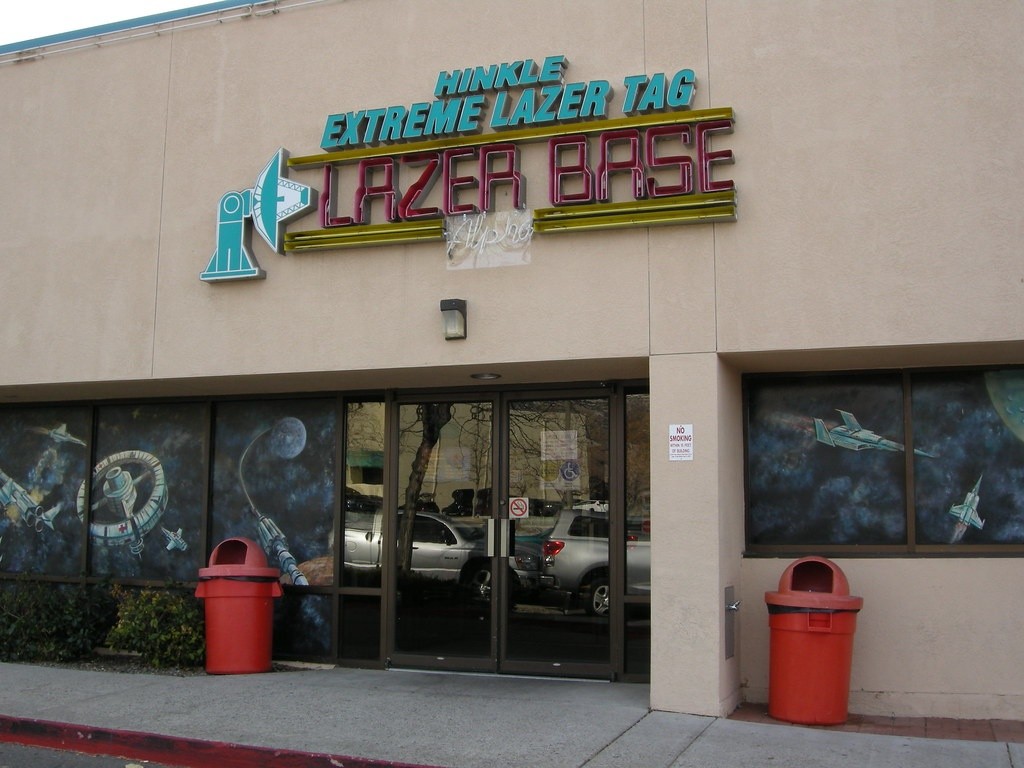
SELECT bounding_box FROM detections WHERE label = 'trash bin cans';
[765,556,863,726]
[195,538,282,675]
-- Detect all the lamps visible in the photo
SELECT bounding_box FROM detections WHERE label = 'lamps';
[441,298,467,340]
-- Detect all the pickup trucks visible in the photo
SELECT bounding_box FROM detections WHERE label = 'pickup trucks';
[342,509,543,611]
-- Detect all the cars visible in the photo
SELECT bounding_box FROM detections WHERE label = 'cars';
[539,509,651,615]
[345,487,361,497]
[442,489,473,514]
[572,500,608,512]
[529,498,561,514]
[347,495,383,510]
[475,488,492,514]
[400,493,439,512]
